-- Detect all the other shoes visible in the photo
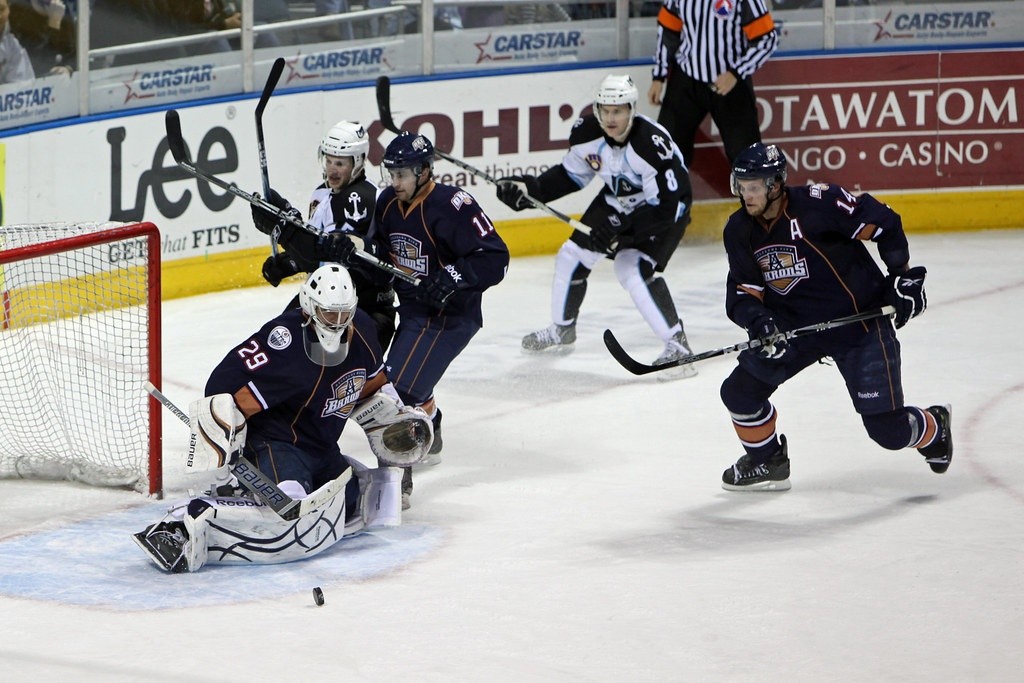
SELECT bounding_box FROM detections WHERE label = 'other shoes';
[130,521,189,573]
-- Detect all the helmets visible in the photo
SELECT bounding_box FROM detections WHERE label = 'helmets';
[298,264,358,368]
[729,142,787,196]
[591,72,639,143]
[317,119,370,195]
[382,130,435,177]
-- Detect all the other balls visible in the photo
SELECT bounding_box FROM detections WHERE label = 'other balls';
[312,586,324,605]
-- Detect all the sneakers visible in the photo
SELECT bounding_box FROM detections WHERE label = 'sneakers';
[400,467,413,510]
[917,403,954,474]
[520,323,577,355]
[651,326,698,382]
[418,408,443,466]
[721,435,791,492]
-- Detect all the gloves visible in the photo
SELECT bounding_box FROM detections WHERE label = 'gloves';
[415,257,480,314]
[262,252,303,287]
[876,265,927,330]
[321,229,379,272]
[250,188,303,244]
[586,218,635,260]
[746,313,792,362]
[495,164,581,211]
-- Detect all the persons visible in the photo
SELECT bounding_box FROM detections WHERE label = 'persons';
[496,73,700,379]
[722,143,953,490]
[648,0,779,163]
[0,0,650,94]
[325,131,510,511]
[250,120,396,358]
[129,257,434,574]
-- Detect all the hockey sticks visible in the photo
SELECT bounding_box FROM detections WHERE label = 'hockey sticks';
[165,108,421,288]
[374,76,621,251]
[254,57,287,256]
[140,379,353,521]
[602,304,897,376]
